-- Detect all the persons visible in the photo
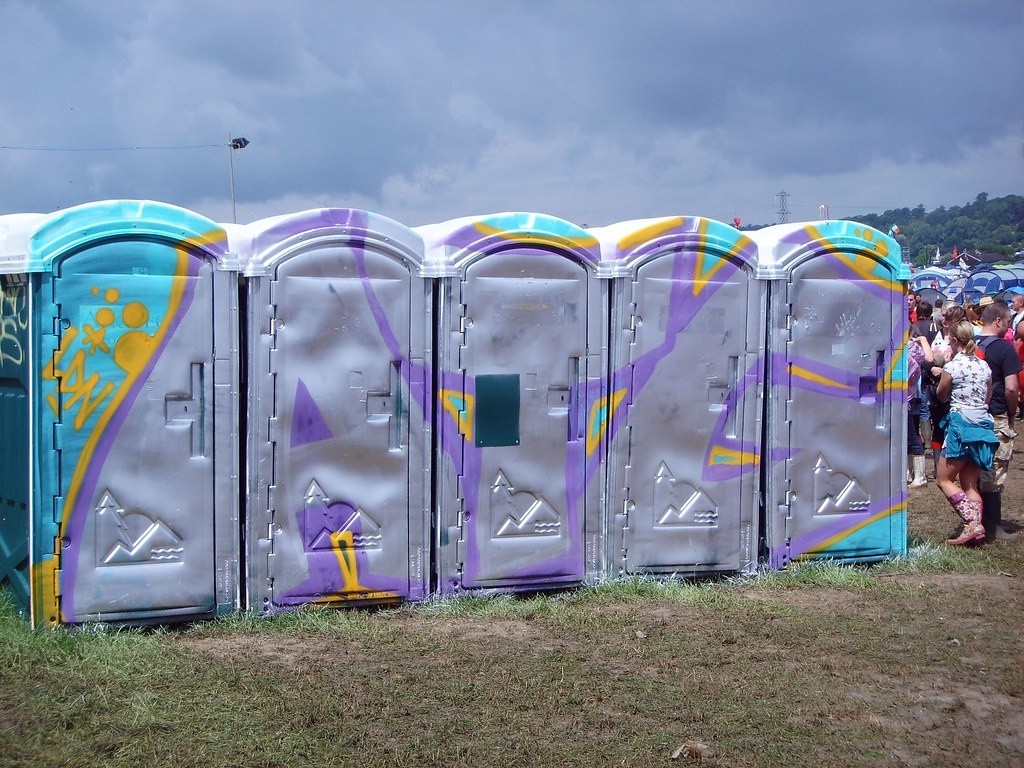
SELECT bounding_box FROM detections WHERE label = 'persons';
[908,287,1024,543]
[935,321,1000,546]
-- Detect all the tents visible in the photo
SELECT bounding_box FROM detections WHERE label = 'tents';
[910,259,1024,304]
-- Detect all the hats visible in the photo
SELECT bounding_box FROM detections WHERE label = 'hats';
[979,297,994,306]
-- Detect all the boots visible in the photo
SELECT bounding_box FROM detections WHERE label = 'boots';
[980,491,1020,544]
[929,442,942,479]
[920,420,933,455]
[947,489,986,545]
[907,454,928,489]
